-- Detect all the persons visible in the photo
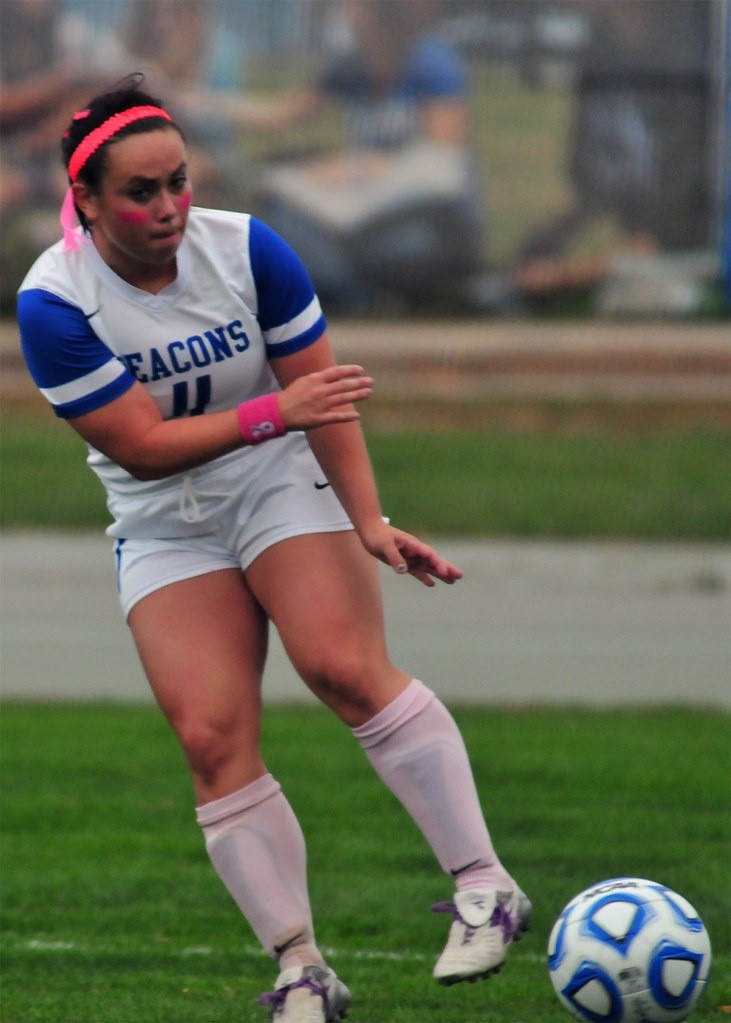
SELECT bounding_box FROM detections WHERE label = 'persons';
[178,0,484,319]
[0,0,176,310]
[17,71,535,1023]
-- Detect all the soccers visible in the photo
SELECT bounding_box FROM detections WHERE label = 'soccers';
[543,875,714,1021]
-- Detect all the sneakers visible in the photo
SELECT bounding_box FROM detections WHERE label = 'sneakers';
[429,879,537,985]
[269,964,350,1023]
